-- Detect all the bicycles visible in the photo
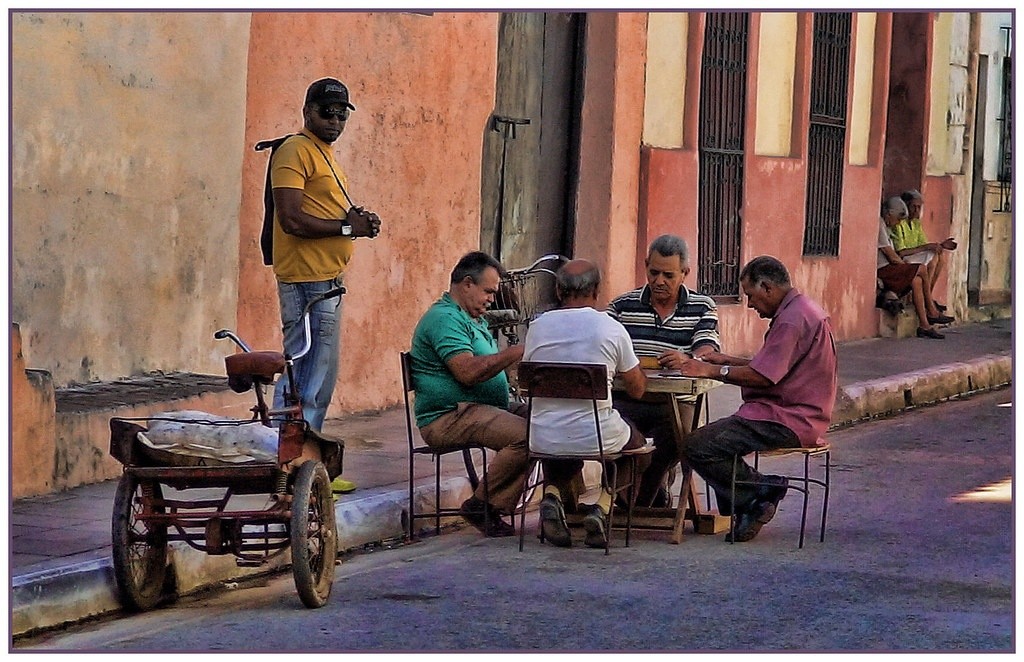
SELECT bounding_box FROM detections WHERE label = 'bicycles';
[464,253,571,512]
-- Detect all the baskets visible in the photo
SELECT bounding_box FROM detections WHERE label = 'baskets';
[484,274,538,330]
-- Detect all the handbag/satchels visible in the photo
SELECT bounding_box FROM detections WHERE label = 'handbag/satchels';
[253,133,361,266]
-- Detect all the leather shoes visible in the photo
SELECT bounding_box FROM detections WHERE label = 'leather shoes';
[725,497,775,542]
[755,475,789,508]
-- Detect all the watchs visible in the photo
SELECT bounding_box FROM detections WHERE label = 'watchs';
[340,219,353,236]
[720,365,730,383]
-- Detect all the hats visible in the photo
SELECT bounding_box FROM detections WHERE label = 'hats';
[305,78,356,111]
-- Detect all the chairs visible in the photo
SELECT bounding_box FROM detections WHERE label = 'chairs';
[399,349,515,542]
[517,360,636,556]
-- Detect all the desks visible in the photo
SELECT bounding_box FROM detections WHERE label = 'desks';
[535,369,726,544]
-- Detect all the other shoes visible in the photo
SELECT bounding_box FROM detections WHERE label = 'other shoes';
[650,466,677,508]
[331,477,357,492]
[333,493,338,502]
[540,493,572,547]
[583,504,608,548]
[934,301,947,311]
[460,494,505,536]
[927,313,955,325]
[917,327,945,340]
[492,511,515,535]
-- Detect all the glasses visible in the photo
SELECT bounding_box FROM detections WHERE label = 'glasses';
[308,105,350,121]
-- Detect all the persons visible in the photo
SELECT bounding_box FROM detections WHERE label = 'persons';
[521,259,647,547]
[679,255,838,542]
[876,190,955,339]
[606,234,721,511]
[409,251,538,538]
[271,77,381,502]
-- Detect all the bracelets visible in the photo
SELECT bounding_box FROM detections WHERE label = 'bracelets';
[685,350,697,360]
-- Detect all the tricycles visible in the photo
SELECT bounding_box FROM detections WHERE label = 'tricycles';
[108,286,346,614]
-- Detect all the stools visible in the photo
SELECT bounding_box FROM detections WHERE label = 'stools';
[730,443,831,549]
